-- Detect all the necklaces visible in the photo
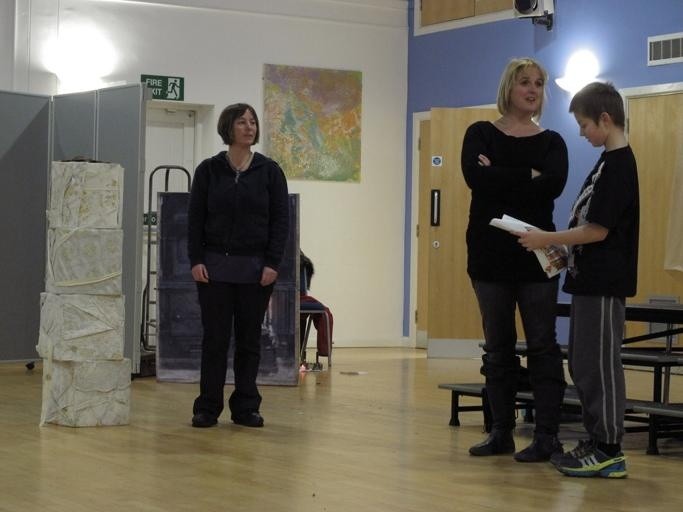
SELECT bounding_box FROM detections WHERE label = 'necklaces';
[225,149,252,174]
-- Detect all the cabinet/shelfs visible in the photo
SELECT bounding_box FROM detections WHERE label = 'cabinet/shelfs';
[420,0,512,27]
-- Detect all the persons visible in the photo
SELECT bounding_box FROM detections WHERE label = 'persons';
[506,80,640,482]
[185,102,291,427]
[459,56,570,464]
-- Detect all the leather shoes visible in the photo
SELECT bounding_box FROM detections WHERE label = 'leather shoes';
[192,412,264,427]
[514,439,563,462]
[468,432,515,456]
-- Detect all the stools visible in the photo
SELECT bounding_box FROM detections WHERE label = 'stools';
[299,302,332,372]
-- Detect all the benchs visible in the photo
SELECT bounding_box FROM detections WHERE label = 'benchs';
[439,299,683,456]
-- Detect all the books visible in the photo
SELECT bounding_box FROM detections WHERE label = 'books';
[487,214,570,279]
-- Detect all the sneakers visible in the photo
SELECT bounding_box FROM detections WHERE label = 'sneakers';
[550,441,628,479]
